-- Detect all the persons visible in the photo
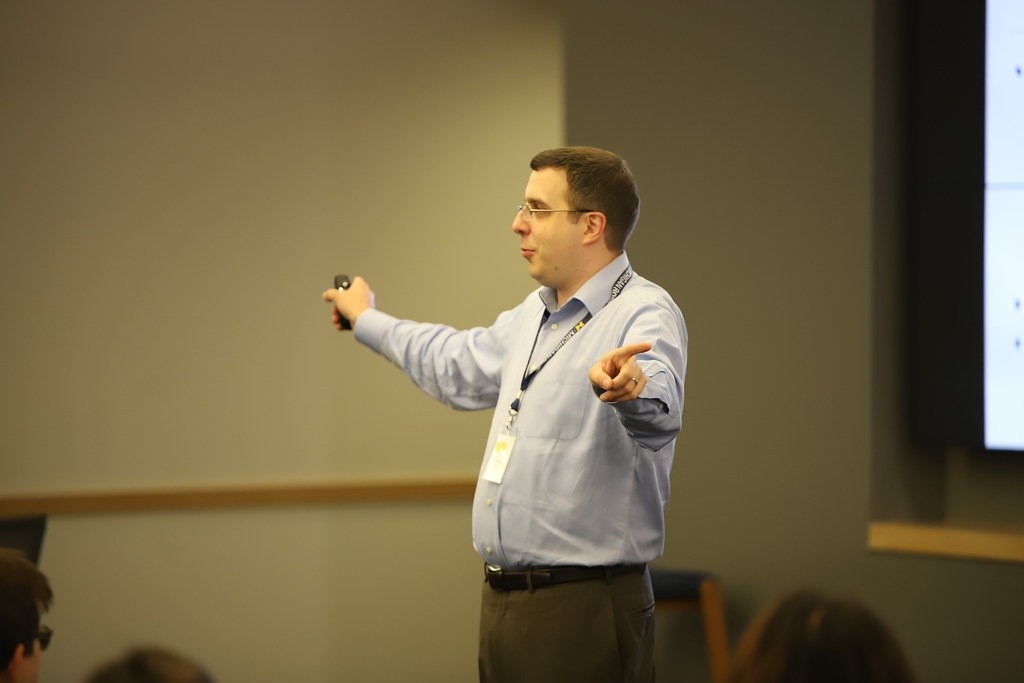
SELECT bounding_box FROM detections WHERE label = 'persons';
[752,590,909,683]
[323,146,686,683]
[0,553,55,683]
[77,644,221,683]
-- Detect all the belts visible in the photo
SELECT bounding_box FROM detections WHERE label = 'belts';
[484,561,645,592]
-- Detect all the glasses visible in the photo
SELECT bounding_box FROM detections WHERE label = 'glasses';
[519,204,594,219]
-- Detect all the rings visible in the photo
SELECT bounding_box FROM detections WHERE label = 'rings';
[632,378,638,385]
